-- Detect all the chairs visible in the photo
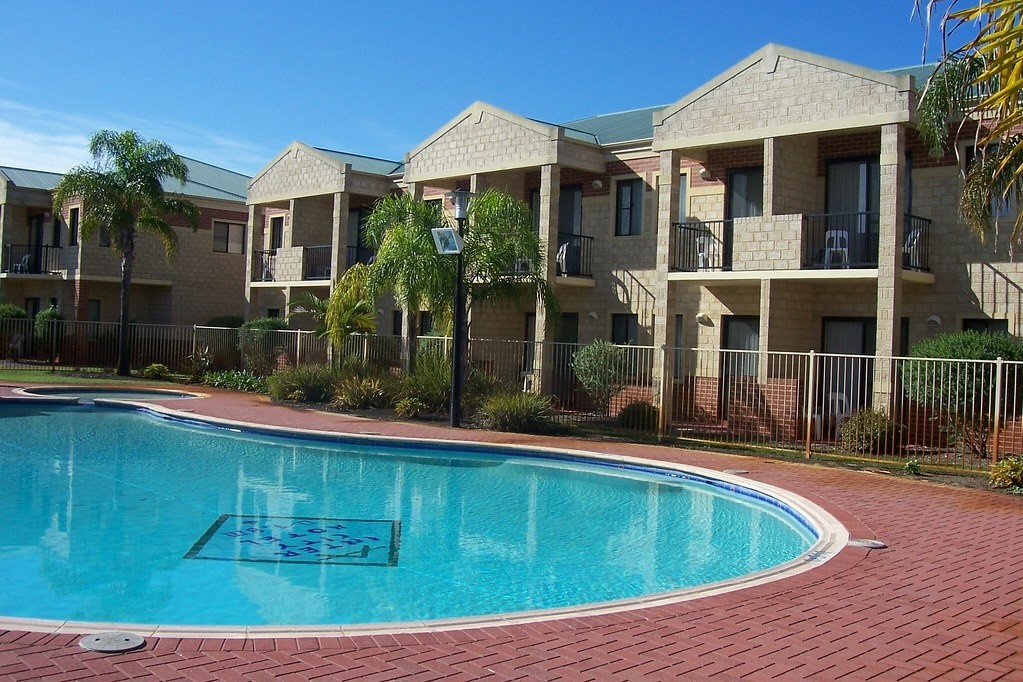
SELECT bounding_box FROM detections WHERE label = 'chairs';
[900,226,924,270]
[696,236,723,273]
[827,392,855,440]
[555,241,570,277]
[824,229,851,269]
[12,255,31,274]
[8,333,26,359]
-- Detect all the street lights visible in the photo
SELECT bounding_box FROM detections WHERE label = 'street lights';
[445,188,472,429]
